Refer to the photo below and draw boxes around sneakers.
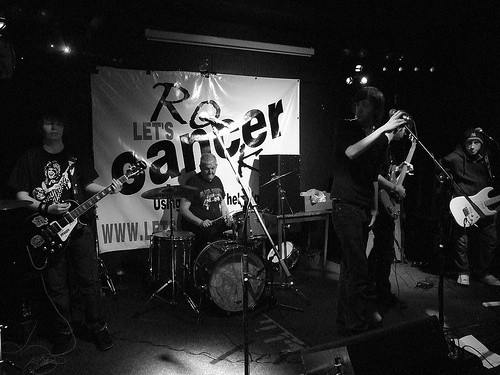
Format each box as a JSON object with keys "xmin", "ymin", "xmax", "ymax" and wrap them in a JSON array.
[
  {"xmin": 482, "ymin": 275, "xmax": 500, "ymax": 286},
  {"xmin": 457, "ymin": 274, "xmax": 469, "ymax": 285}
]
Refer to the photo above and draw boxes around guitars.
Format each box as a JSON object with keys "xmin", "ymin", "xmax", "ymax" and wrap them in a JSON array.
[
  {"xmin": 448, "ymin": 186, "xmax": 500, "ymax": 229},
  {"xmin": 22, "ymin": 160, "xmax": 147, "ymax": 259},
  {"xmin": 379, "ymin": 133, "xmax": 417, "ymax": 220}
]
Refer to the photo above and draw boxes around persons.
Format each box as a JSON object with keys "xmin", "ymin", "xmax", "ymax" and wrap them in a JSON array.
[
  {"xmin": 330, "ymin": 87, "xmax": 407, "ymax": 336},
  {"xmin": 435, "ymin": 130, "xmax": 500, "ymax": 286},
  {"xmin": 13, "ymin": 109, "xmax": 121, "ymax": 354},
  {"xmin": 368, "ymin": 115, "xmax": 417, "ymax": 310},
  {"xmin": 179, "ymin": 153, "xmax": 236, "ymax": 246}
]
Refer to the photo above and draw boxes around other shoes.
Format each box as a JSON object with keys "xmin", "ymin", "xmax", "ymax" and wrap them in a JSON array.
[
  {"xmin": 91, "ymin": 322, "xmax": 114, "ymax": 351},
  {"xmin": 50, "ymin": 331, "xmax": 79, "ymax": 356},
  {"xmin": 378, "ymin": 293, "xmax": 408, "ymax": 310}
]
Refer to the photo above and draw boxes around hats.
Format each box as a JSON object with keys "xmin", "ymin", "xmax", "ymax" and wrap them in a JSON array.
[{"xmin": 463, "ymin": 130, "xmax": 484, "ymax": 143}]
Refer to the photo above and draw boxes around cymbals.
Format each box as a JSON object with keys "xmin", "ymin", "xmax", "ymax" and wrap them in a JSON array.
[
  {"xmin": 260, "ymin": 170, "xmax": 295, "ymax": 189},
  {"xmin": 141, "ymin": 184, "xmax": 199, "ymax": 199},
  {"xmin": 1, "ymin": 198, "xmax": 33, "ymax": 210}
]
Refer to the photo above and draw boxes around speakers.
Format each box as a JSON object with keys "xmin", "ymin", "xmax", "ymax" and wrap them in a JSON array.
[
  {"xmin": 259, "ymin": 154, "xmax": 305, "ymax": 215},
  {"xmin": 301, "ymin": 314, "xmax": 459, "ymax": 375},
  {"xmin": 0, "ymin": 198, "xmax": 34, "ymax": 325}
]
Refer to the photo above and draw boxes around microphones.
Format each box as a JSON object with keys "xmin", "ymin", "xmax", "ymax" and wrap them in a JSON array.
[
  {"xmin": 475, "ymin": 128, "xmax": 494, "ymax": 141},
  {"xmin": 199, "ymin": 112, "xmax": 229, "ymax": 129},
  {"xmin": 389, "ymin": 109, "xmax": 411, "ymax": 120}
]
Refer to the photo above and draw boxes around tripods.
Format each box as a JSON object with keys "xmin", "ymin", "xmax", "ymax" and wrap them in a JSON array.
[
  {"xmin": 265, "ymin": 179, "xmax": 313, "ymax": 307},
  {"xmin": 132, "ymin": 197, "xmax": 204, "ymax": 326}
]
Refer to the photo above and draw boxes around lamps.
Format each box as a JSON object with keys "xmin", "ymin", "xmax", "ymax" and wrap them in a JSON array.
[{"xmin": 343, "ymin": 56, "xmax": 435, "ymax": 86}]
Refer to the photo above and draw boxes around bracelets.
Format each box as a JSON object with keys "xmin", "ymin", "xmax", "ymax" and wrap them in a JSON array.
[
  {"xmin": 44, "ymin": 203, "xmax": 51, "ymax": 211},
  {"xmin": 38, "ymin": 202, "xmax": 46, "ymax": 210}
]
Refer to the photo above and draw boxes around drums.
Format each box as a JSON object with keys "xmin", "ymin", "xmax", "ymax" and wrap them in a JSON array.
[
  {"xmin": 152, "ymin": 231, "xmax": 195, "ymax": 272},
  {"xmin": 267, "ymin": 240, "xmax": 299, "ymax": 273},
  {"xmin": 193, "ymin": 239, "xmax": 267, "ymax": 312},
  {"xmin": 233, "ymin": 209, "xmax": 268, "ymax": 241}
]
